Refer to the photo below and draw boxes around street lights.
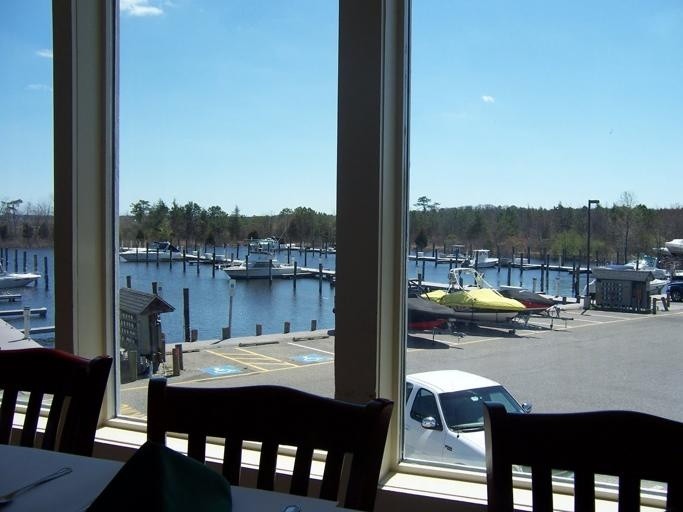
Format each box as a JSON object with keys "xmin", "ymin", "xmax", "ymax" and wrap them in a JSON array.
[{"xmin": 586, "ymin": 200, "xmax": 599, "ymax": 296}]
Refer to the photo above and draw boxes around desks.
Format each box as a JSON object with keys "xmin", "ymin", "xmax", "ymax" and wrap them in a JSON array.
[{"xmin": 2, "ymin": 445, "xmax": 353, "ymax": 511}]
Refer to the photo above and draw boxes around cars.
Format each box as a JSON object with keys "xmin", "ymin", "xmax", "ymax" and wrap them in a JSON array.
[{"xmin": 404, "ymin": 370, "xmax": 533, "ymax": 466}]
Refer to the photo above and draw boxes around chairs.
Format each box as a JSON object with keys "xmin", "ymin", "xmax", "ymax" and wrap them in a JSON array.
[
  {"xmin": 146, "ymin": 374, "xmax": 395, "ymax": 511},
  {"xmin": 0, "ymin": 346, "xmax": 115, "ymax": 457},
  {"xmin": 483, "ymin": 400, "xmax": 682, "ymax": 511}
]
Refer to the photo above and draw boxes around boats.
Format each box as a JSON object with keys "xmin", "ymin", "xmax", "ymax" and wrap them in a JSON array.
[
  {"xmin": 407, "ymin": 268, "xmax": 556, "ymax": 331},
  {"xmin": 0, "ymin": 274, "xmax": 42, "ymax": 290},
  {"xmin": 223, "ymin": 262, "xmax": 302, "ymax": 279},
  {"xmin": 469, "ymin": 249, "xmax": 499, "ymax": 268},
  {"xmin": 119, "ymin": 250, "xmax": 184, "ymax": 263}
]
